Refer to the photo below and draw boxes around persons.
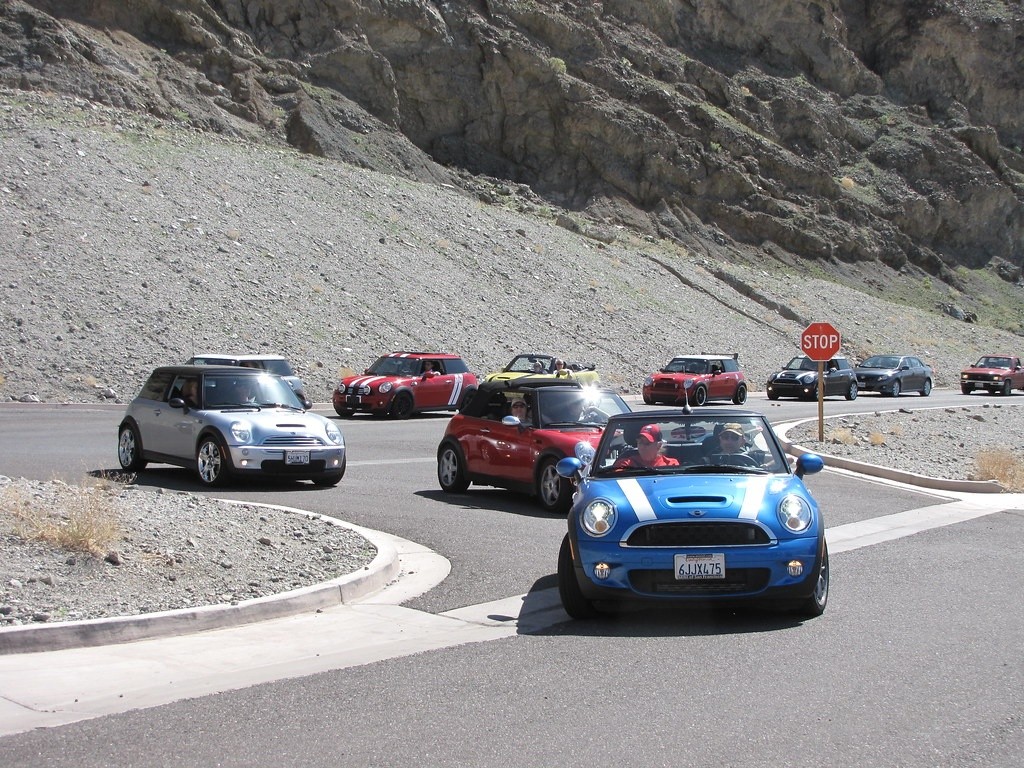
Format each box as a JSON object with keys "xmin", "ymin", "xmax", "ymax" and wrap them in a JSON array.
[
  {"xmin": 613, "ymin": 425, "xmax": 680, "ymax": 469},
  {"xmin": 232, "ymin": 384, "xmax": 257, "ymax": 402},
  {"xmin": 533, "ymin": 362, "xmax": 547, "ymax": 374},
  {"xmin": 700, "ymin": 423, "xmax": 750, "ymax": 466},
  {"xmin": 564, "ymin": 397, "xmax": 585, "ymax": 425},
  {"xmin": 419, "ymin": 361, "xmax": 442, "ymax": 376},
  {"xmin": 553, "ymin": 359, "xmax": 565, "ymax": 375},
  {"xmin": 511, "ymin": 397, "xmax": 528, "ymax": 423},
  {"xmin": 184, "ymin": 381, "xmax": 201, "ymax": 409}
]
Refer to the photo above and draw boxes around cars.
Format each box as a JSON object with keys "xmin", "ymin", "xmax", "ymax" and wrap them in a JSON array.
[
  {"xmin": 118, "ymin": 365, "xmax": 346, "ymax": 487},
  {"xmin": 436, "ymin": 378, "xmax": 634, "ymax": 517},
  {"xmin": 853, "ymin": 355, "xmax": 936, "ymax": 397},
  {"xmin": 333, "ymin": 352, "xmax": 477, "ymax": 418},
  {"xmin": 643, "ymin": 352, "xmax": 746, "ymax": 404},
  {"xmin": 557, "ymin": 392, "xmax": 829, "ymax": 616},
  {"xmin": 960, "ymin": 355, "xmax": 1024, "ymax": 397},
  {"xmin": 766, "ymin": 357, "xmax": 858, "ymax": 401},
  {"xmin": 185, "ymin": 354, "xmax": 305, "ymax": 410}
]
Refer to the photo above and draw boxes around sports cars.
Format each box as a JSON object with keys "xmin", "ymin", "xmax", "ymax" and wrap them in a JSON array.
[{"xmin": 482, "ymin": 354, "xmax": 601, "ymax": 388}]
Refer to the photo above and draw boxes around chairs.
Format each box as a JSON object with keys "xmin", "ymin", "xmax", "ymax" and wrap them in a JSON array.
[
  {"xmin": 701, "ymin": 424, "xmax": 722, "ymax": 456},
  {"xmin": 484, "ymin": 393, "xmax": 508, "ymax": 421},
  {"xmin": 711, "ymin": 365, "xmax": 718, "ymax": 373},
  {"xmin": 432, "ymin": 362, "xmax": 444, "ymax": 376},
  {"xmin": 409, "ymin": 362, "xmax": 418, "ymax": 372},
  {"xmin": 690, "ymin": 364, "xmax": 697, "ymax": 372},
  {"xmin": 618, "ymin": 424, "xmax": 640, "ymax": 458}
]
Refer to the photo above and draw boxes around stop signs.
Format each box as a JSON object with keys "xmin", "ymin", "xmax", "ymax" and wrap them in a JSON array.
[{"xmin": 799, "ymin": 322, "xmax": 840, "ymax": 363}]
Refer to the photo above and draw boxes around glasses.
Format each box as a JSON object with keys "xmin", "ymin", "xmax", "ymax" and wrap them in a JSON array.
[
  {"xmin": 534, "ymin": 365, "xmax": 540, "ymax": 368},
  {"xmin": 719, "ymin": 432, "xmax": 741, "ymax": 440},
  {"xmin": 512, "ymin": 404, "xmax": 524, "ymax": 409},
  {"xmin": 557, "ymin": 363, "xmax": 564, "ymax": 366},
  {"xmin": 424, "ymin": 363, "xmax": 431, "ymax": 366},
  {"xmin": 636, "ymin": 438, "xmax": 661, "ymax": 446}
]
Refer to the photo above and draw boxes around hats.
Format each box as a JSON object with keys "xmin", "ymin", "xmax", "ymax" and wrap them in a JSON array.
[
  {"xmin": 638, "ymin": 424, "xmax": 662, "ymax": 443},
  {"xmin": 718, "ymin": 423, "xmax": 745, "ymax": 439},
  {"xmin": 510, "ymin": 397, "xmax": 527, "ymax": 407}
]
